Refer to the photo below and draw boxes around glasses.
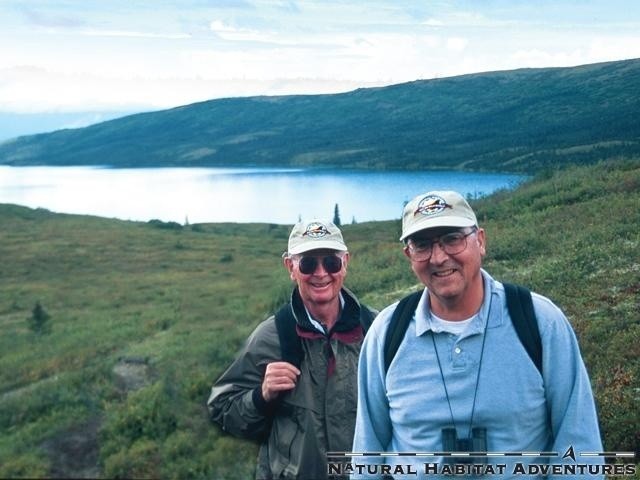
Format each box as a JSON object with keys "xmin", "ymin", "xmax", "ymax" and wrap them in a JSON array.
[
  {"xmin": 406, "ymin": 229, "xmax": 476, "ymax": 261},
  {"xmin": 291, "ymin": 256, "xmax": 344, "ymax": 274}
]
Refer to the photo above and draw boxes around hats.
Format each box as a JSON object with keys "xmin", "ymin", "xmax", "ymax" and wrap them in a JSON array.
[
  {"xmin": 398, "ymin": 190, "xmax": 478, "ymax": 244},
  {"xmin": 287, "ymin": 216, "xmax": 348, "ymax": 256}
]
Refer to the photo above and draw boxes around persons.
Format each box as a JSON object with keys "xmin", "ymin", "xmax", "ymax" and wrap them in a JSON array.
[
  {"xmin": 206, "ymin": 216, "xmax": 381, "ymax": 480},
  {"xmin": 351, "ymin": 192, "xmax": 605, "ymax": 480}
]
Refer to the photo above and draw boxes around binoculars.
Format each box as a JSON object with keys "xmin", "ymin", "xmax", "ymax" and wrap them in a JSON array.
[{"xmin": 442, "ymin": 427, "xmax": 488, "ymax": 476}]
